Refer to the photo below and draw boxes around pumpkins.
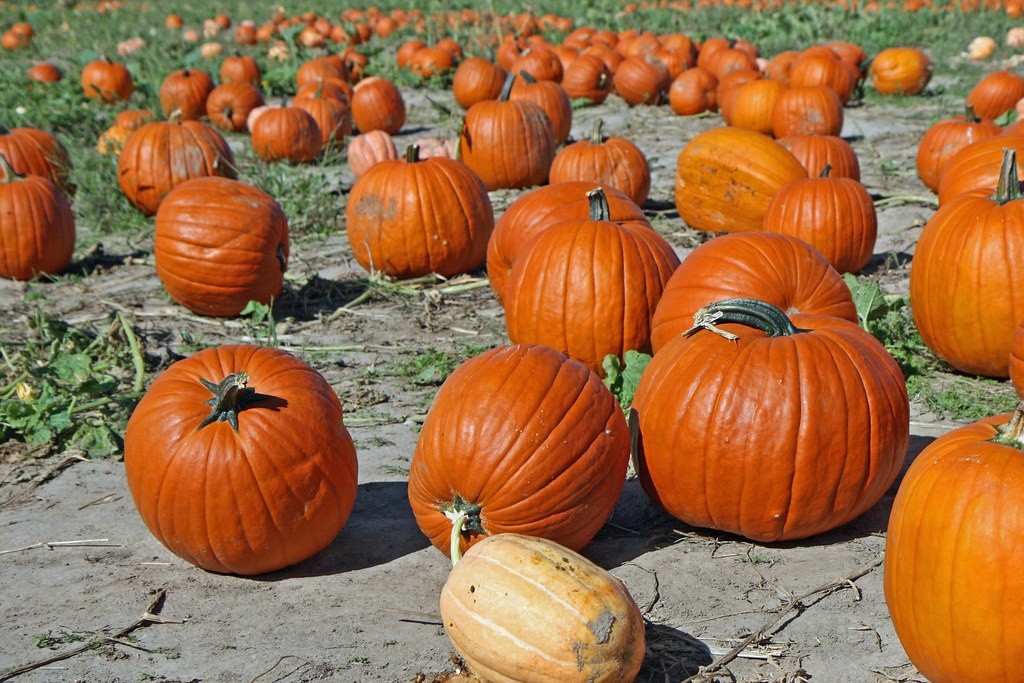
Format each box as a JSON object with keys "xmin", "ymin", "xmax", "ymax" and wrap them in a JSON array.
[{"xmin": 0, "ymin": 0, "xmax": 1024, "ymax": 683}]
